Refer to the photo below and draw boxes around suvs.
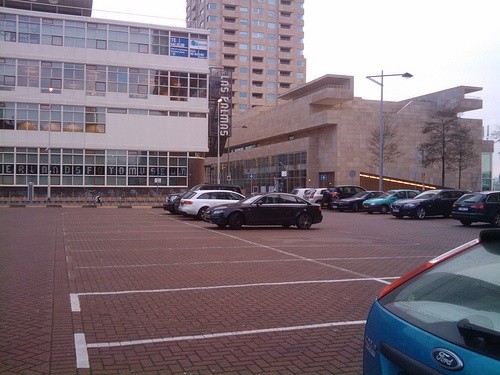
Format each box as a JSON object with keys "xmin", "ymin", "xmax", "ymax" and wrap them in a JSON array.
[{"xmin": 162, "ymin": 184, "xmax": 241, "ymax": 214}]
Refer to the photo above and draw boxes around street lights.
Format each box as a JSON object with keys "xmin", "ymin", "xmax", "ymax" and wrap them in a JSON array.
[
  {"xmin": 216, "ymin": 97, "xmax": 225, "ymax": 182},
  {"xmin": 226, "ymin": 125, "xmax": 248, "ymax": 184},
  {"xmin": 366, "ymin": 71, "xmax": 414, "ymax": 190}
]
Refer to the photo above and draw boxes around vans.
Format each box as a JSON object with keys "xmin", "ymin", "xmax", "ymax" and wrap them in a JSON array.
[{"xmin": 323, "ymin": 185, "xmax": 367, "ymax": 210}]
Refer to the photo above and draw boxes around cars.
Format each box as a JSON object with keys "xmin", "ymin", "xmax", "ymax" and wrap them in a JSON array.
[
  {"xmin": 205, "ymin": 193, "xmax": 323, "ymax": 230},
  {"xmin": 389, "ymin": 189, "xmax": 473, "ymax": 220},
  {"xmin": 291, "ymin": 188, "xmax": 311, "ymax": 200},
  {"xmin": 361, "ymin": 189, "xmax": 421, "ymax": 214},
  {"xmin": 331, "ymin": 191, "xmax": 390, "ymax": 213},
  {"xmin": 303, "ymin": 187, "xmax": 328, "ymax": 209},
  {"xmin": 178, "ymin": 189, "xmax": 246, "ymax": 222},
  {"xmin": 362, "ymin": 215, "xmax": 500, "ymax": 375},
  {"xmin": 451, "ymin": 190, "xmax": 500, "ymax": 228}
]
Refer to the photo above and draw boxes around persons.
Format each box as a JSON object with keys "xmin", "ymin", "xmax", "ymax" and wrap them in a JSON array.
[{"xmin": 322, "ymin": 182, "xmax": 340, "ymax": 209}]
